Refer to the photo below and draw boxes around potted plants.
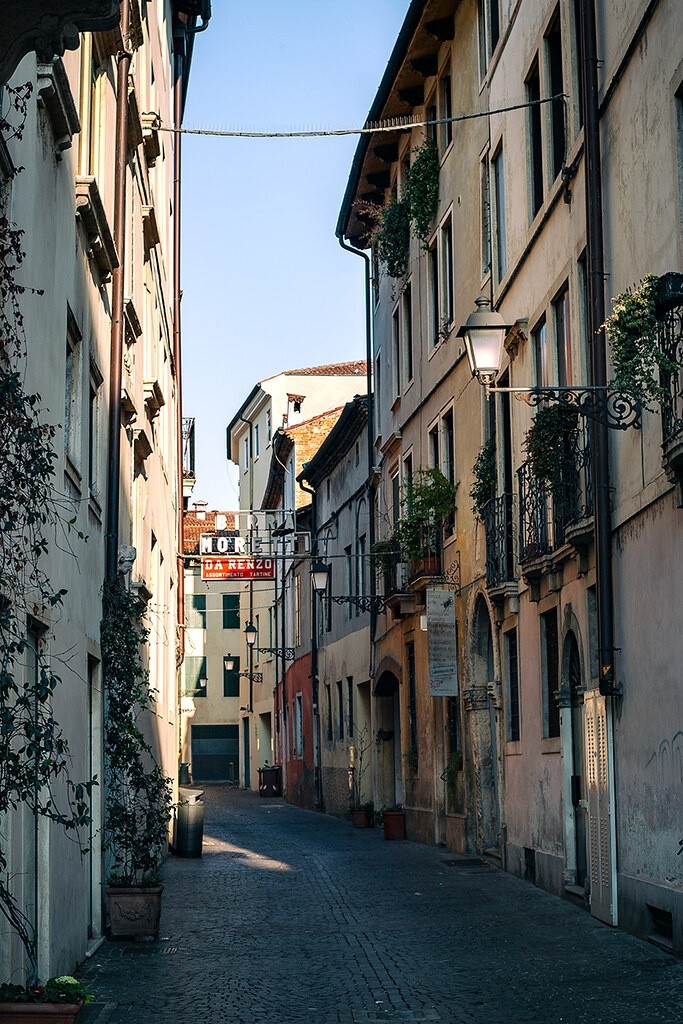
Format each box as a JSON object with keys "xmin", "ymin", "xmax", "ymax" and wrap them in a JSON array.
[
  {"xmin": 381, "ymin": 804, "xmax": 406, "ymax": 841},
  {"xmin": 100, "ymin": 777, "xmax": 177, "ymax": 939},
  {"xmin": 343, "ymin": 724, "xmax": 377, "ymax": 829}
]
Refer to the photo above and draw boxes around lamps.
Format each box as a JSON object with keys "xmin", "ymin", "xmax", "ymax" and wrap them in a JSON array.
[
  {"xmin": 180, "ymin": 674, "xmax": 208, "ymax": 696},
  {"xmin": 242, "ymin": 621, "xmax": 296, "ymax": 661},
  {"xmin": 455, "ymin": 295, "xmax": 644, "ymax": 433},
  {"xmin": 308, "ymin": 557, "xmax": 390, "ymax": 616},
  {"xmin": 224, "ymin": 653, "xmax": 263, "ymax": 683}
]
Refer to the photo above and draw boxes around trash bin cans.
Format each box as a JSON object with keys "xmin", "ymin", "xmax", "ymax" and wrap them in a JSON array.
[
  {"xmin": 179, "ymin": 762, "xmax": 191, "ymax": 785},
  {"xmin": 177, "ymin": 789, "xmax": 205, "ymax": 858},
  {"xmin": 256, "ymin": 764, "xmax": 282, "ymax": 798}
]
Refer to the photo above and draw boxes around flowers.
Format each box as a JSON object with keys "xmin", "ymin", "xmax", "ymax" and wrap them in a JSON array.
[{"xmin": 1, "ymin": 967, "xmax": 103, "ymax": 1007}]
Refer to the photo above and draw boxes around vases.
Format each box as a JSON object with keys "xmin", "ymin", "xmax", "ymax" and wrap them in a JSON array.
[{"xmin": 0, "ymin": 1000, "xmax": 82, "ymax": 1024}]
